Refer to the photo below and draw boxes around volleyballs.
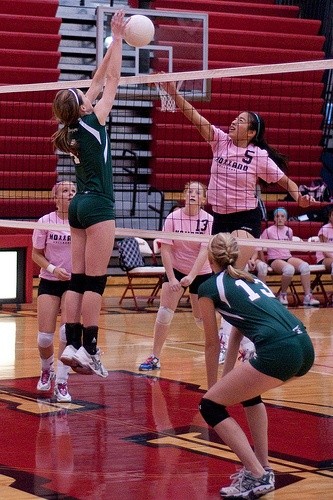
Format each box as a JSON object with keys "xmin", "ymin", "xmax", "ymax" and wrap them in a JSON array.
[{"xmin": 123, "ymin": 15, "xmax": 155, "ymax": 47}]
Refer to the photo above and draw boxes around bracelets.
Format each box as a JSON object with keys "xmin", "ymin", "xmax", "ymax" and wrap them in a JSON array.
[{"xmin": 46, "ymin": 264, "xmax": 57, "ymax": 275}]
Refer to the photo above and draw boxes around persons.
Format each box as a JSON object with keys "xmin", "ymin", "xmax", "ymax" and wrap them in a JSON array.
[
  {"xmin": 31, "ymin": 181, "xmax": 76, "ymax": 401},
  {"xmin": 316, "ymin": 208, "xmax": 333, "ymax": 279},
  {"xmin": 198, "ymin": 232, "xmax": 315, "ymax": 499},
  {"xmin": 244, "ymin": 248, "xmax": 267, "ymax": 284},
  {"xmin": 156, "ymin": 71, "xmax": 320, "ymax": 365},
  {"xmin": 260, "ymin": 208, "xmax": 320, "ymax": 306},
  {"xmin": 138, "ymin": 182, "xmax": 214, "ymax": 370},
  {"xmin": 52, "ymin": 10, "xmax": 131, "ymax": 378}
]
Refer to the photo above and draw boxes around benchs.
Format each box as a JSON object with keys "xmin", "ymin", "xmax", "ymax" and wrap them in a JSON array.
[
  {"xmin": 0, "ymin": 0, "xmax": 59, "ymax": 219},
  {"xmin": 128, "ymin": 0, "xmax": 333, "ymax": 267}
]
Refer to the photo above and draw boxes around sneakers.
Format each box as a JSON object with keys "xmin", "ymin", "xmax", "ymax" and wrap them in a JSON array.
[
  {"xmin": 279, "ymin": 291, "xmax": 289, "ymax": 305},
  {"xmin": 60, "ymin": 345, "xmax": 95, "ymax": 375},
  {"xmin": 73, "ymin": 346, "xmax": 108, "ymax": 378},
  {"xmin": 239, "ymin": 351, "xmax": 257, "ymax": 363},
  {"xmin": 219, "ymin": 334, "xmax": 229, "ymax": 364},
  {"xmin": 139, "ymin": 356, "xmax": 160, "ymax": 370},
  {"xmin": 219, "ymin": 468, "xmax": 275, "ymax": 500},
  {"xmin": 303, "ymin": 295, "xmax": 320, "ymax": 305},
  {"xmin": 36, "ymin": 366, "xmax": 56, "ymax": 391},
  {"xmin": 53, "ymin": 379, "xmax": 71, "ymax": 402}
]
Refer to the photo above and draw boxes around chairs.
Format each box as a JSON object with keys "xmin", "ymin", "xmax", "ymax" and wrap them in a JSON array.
[{"xmin": 117, "ymin": 233, "xmax": 333, "ymax": 308}]
